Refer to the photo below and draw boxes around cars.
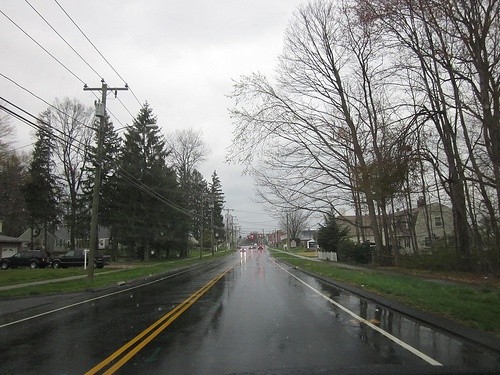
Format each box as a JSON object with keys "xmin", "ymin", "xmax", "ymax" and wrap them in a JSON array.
[
  {"xmin": 258, "ymin": 245, "xmax": 263, "ymax": 250},
  {"xmin": 240, "ymin": 247, "xmax": 247, "ymax": 252}
]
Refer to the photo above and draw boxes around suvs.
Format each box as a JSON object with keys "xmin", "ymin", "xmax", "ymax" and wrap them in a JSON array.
[
  {"xmin": 0, "ymin": 250, "xmax": 49, "ymax": 269},
  {"xmin": 52, "ymin": 248, "xmax": 104, "ymax": 269}
]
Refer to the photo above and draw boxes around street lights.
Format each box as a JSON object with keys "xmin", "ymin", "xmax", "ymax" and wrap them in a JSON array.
[{"xmin": 80, "ymin": 151, "xmax": 103, "ymax": 281}]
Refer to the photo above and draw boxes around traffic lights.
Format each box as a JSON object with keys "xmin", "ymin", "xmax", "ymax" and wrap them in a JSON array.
[{"xmin": 252, "ymin": 235, "xmax": 254, "ymax": 239}]
